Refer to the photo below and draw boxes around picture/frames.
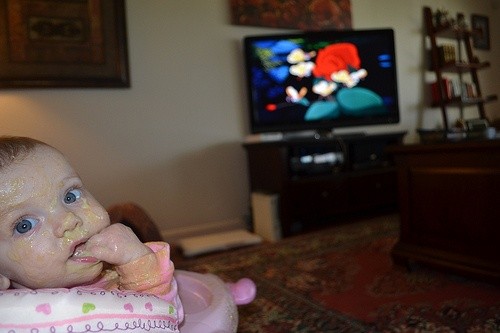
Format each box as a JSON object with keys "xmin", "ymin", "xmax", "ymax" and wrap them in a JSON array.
[
  {"xmin": 470, "ymin": 13, "xmax": 490, "ymax": 50},
  {"xmin": 0, "ymin": 0, "xmax": 130, "ymax": 89}
]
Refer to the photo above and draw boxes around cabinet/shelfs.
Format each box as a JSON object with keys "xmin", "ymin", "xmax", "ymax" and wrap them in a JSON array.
[
  {"xmin": 424, "ymin": 6, "xmax": 500, "ymax": 142},
  {"xmin": 243, "ymin": 131, "xmax": 410, "ymax": 238}
]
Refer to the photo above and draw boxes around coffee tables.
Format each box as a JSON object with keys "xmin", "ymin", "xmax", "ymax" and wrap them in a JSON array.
[{"xmin": 387, "ymin": 139, "xmax": 500, "ymax": 283}]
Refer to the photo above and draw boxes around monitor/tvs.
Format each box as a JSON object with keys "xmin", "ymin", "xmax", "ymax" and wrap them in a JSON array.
[{"xmin": 244, "ymin": 27, "xmax": 400, "ymax": 141}]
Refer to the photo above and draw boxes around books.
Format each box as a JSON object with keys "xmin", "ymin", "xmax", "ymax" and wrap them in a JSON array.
[
  {"xmin": 437, "ymin": 45, "xmax": 456, "ymax": 62},
  {"xmin": 432, "ymin": 80, "xmax": 474, "ymax": 104}
]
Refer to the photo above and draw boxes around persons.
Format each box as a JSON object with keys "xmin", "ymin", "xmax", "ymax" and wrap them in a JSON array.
[{"xmin": 0, "ymin": 135, "xmax": 185, "ymax": 333}]
